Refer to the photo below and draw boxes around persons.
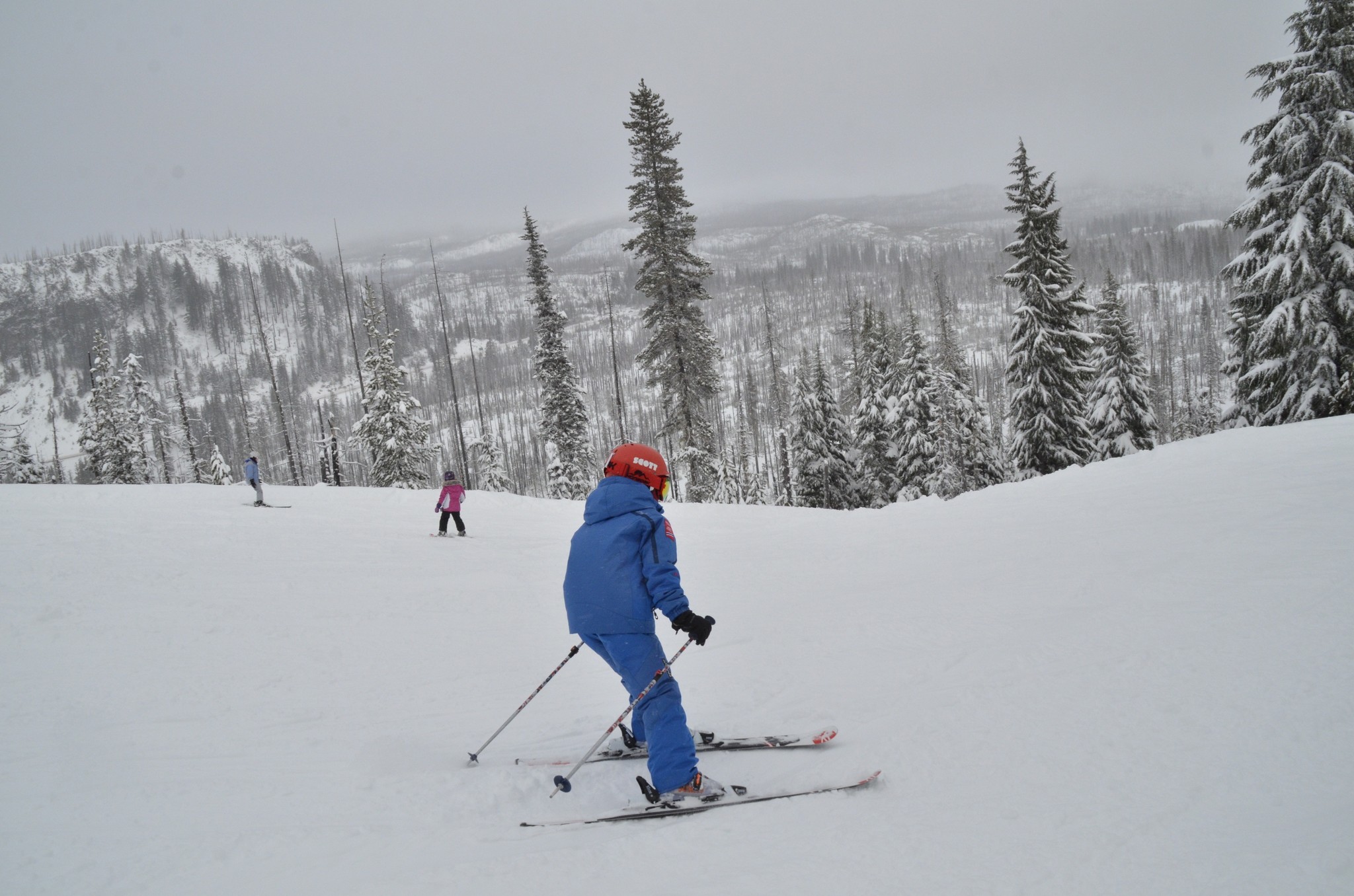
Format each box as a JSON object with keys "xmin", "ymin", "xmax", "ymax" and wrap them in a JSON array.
[
  {"xmin": 435, "ymin": 471, "xmax": 466, "ymax": 536},
  {"xmin": 563, "ymin": 443, "xmax": 723, "ymax": 797},
  {"xmin": 244, "ymin": 451, "xmax": 267, "ymax": 507}
]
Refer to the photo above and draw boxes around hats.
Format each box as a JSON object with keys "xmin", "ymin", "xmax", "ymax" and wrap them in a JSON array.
[{"xmin": 250, "ymin": 452, "xmax": 260, "ymax": 458}]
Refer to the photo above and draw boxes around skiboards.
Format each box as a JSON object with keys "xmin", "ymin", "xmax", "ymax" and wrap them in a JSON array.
[
  {"xmin": 429, "ymin": 531, "xmax": 476, "ymax": 538},
  {"xmin": 517, "ymin": 725, "xmax": 883, "ymax": 829},
  {"xmin": 241, "ymin": 502, "xmax": 292, "ymax": 508}
]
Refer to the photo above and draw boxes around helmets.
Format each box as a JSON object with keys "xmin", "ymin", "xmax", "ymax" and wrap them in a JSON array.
[
  {"xmin": 604, "ymin": 444, "xmax": 669, "ymax": 502},
  {"xmin": 444, "ymin": 471, "xmax": 455, "ymax": 480}
]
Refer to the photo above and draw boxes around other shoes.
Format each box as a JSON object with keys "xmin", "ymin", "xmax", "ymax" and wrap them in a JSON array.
[
  {"xmin": 436, "ymin": 531, "xmax": 447, "ymax": 537},
  {"xmin": 456, "ymin": 531, "xmax": 465, "ymax": 536}
]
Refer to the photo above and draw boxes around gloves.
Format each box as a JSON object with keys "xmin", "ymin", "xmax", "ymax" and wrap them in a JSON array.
[
  {"xmin": 250, "ymin": 479, "xmax": 257, "ymax": 488},
  {"xmin": 435, "ymin": 507, "xmax": 439, "ymax": 513},
  {"xmin": 672, "ymin": 611, "xmax": 715, "ymax": 648},
  {"xmin": 259, "ymin": 479, "xmax": 262, "ymax": 483}
]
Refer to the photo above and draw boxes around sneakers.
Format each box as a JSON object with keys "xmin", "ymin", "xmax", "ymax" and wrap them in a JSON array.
[
  {"xmin": 658, "ymin": 770, "xmax": 723, "ymax": 796},
  {"xmin": 255, "ymin": 502, "xmax": 269, "ymax": 507}
]
